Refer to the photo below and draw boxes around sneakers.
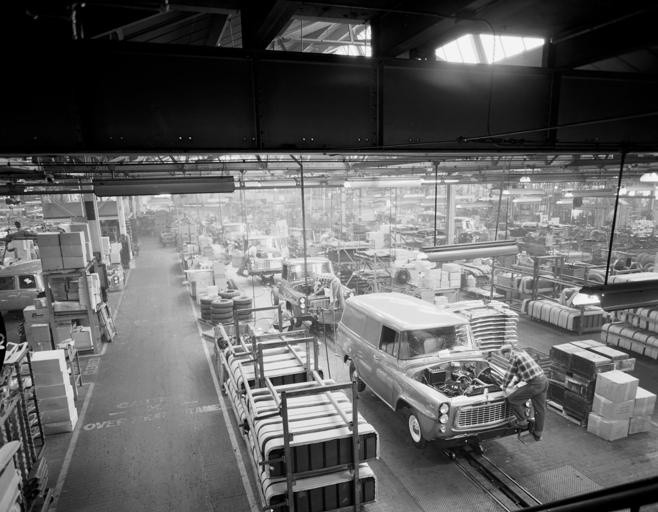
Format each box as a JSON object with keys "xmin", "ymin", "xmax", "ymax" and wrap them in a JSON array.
[
  {"xmin": 529, "ymin": 423, "xmax": 540, "ymax": 441},
  {"xmin": 511, "ymin": 420, "xmax": 529, "ymax": 430}
]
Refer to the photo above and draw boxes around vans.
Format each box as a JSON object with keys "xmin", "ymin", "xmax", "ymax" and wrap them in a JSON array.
[{"xmin": 0, "ymin": 260, "xmax": 46, "ymax": 316}]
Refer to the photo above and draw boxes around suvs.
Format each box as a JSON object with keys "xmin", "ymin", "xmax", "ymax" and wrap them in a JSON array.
[
  {"xmin": 202, "ymin": 211, "xmax": 353, "ymax": 333},
  {"xmin": 334, "ymin": 289, "xmax": 536, "ymax": 453}
]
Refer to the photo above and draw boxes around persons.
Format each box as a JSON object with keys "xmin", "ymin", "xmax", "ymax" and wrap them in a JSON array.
[
  {"xmin": 310, "ymin": 272, "xmax": 345, "ymax": 313},
  {"xmin": 237, "ymin": 240, "xmax": 260, "ymax": 276},
  {"xmin": 498, "ymin": 343, "xmax": 550, "ymax": 441},
  {"xmin": 517, "ymin": 249, "xmax": 534, "ymax": 267}
]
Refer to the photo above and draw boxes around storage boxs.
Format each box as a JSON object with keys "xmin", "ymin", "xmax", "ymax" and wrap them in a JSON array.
[
  {"xmin": 182, "ymin": 261, "xmax": 231, "ymax": 305},
  {"xmin": 427, "ymin": 262, "xmax": 463, "ymax": 293},
  {"xmin": 17, "ymin": 221, "xmax": 123, "ymax": 435},
  {"xmin": 539, "ymin": 335, "xmax": 657, "ymax": 445}
]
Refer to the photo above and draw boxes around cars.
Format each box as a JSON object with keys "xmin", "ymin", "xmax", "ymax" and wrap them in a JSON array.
[{"xmin": 455, "ymin": 218, "xmax": 489, "ymax": 240}]
